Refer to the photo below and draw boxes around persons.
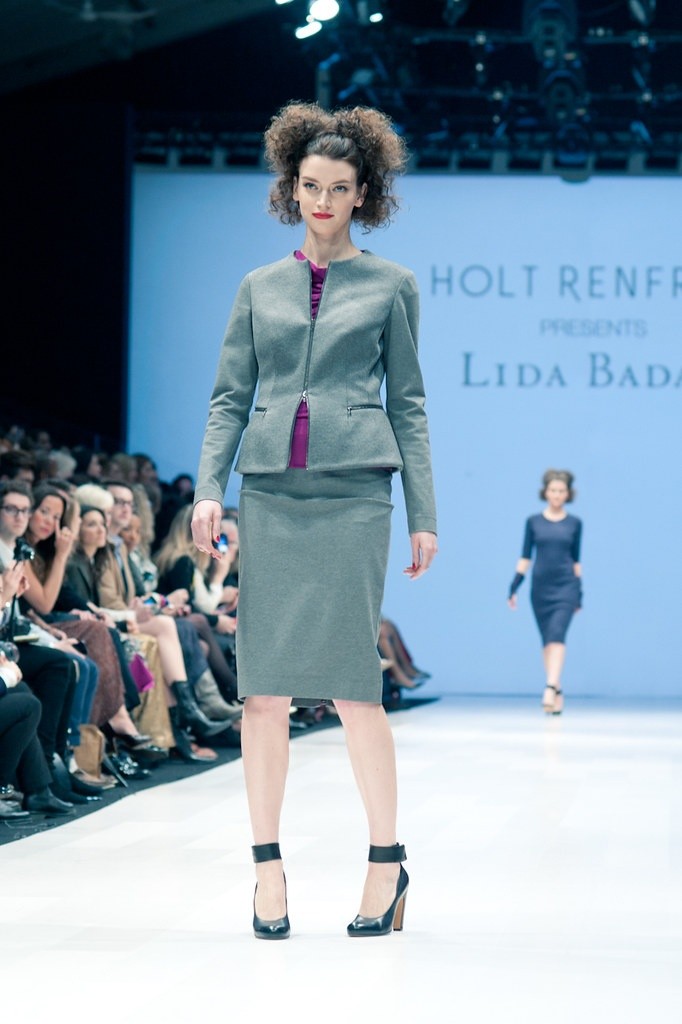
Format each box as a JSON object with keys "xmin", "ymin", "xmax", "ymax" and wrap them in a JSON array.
[
  {"xmin": 191, "ymin": 105, "xmax": 440, "ymax": 938},
  {"xmin": 508, "ymin": 470, "xmax": 582, "ymax": 717},
  {"xmin": 0, "ymin": 427, "xmax": 430, "ymax": 817}
]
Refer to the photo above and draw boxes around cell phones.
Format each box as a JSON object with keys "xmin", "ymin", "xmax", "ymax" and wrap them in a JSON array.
[
  {"xmin": 142, "ymin": 596, "xmax": 157, "ymax": 605},
  {"xmin": 73, "ymin": 640, "xmax": 89, "ymax": 655},
  {"xmin": 213, "ymin": 532, "xmax": 229, "ymax": 554}
]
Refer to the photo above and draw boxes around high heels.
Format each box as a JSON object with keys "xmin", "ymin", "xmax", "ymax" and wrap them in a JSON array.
[
  {"xmin": 347, "ymin": 842, "xmax": 409, "ymax": 936},
  {"xmin": 251, "ymin": 843, "xmax": 290, "ymax": 938}
]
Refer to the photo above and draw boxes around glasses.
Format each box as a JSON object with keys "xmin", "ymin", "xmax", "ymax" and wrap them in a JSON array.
[
  {"xmin": 0, "ymin": 504, "xmax": 33, "ymax": 517},
  {"xmin": 112, "ymin": 498, "xmax": 134, "ymax": 508}
]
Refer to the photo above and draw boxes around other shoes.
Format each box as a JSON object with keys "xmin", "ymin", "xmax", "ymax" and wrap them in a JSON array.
[
  {"xmin": 540, "ymin": 684, "xmax": 564, "ymax": 715},
  {"xmin": 0, "ymin": 722, "xmax": 168, "ymax": 822}
]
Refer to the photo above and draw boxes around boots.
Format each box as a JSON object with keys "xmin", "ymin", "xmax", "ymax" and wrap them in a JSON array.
[{"xmin": 166, "ymin": 671, "xmax": 245, "ymax": 763}]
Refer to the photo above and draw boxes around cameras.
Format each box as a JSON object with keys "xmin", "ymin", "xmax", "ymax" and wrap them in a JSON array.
[
  {"xmin": 0, "ymin": 641, "xmax": 19, "ymax": 664},
  {"xmin": 13, "ymin": 539, "xmax": 34, "ymax": 561}
]
